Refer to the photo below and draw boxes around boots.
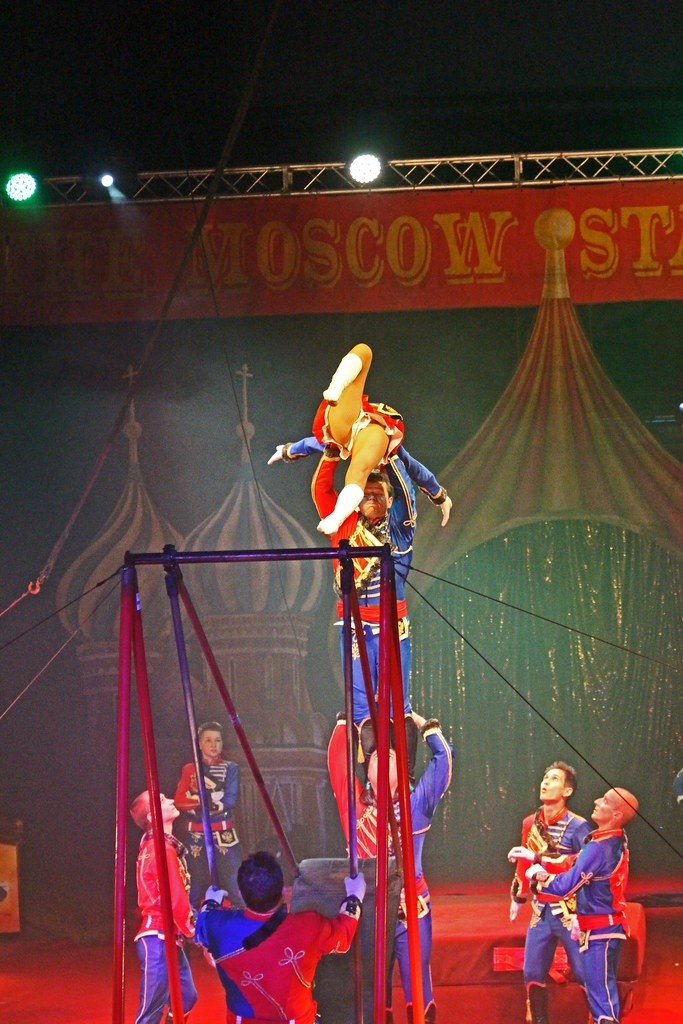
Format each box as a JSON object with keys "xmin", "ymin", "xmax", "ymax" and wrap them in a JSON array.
[
  {"xmin": 406, "ymin": 1000, "xmax": 437, "ymax": 1024},
  {"xmin": 593, "ymin": 1015, "xmax": 619, "ymax": 1024},
  {"xmin": 352, "ymin": 714, "xmax": 418, "ymax": 805},
  {"xmin": 384, "ymin": 1007, "xmax": 394, "ymax": 1024},
  {"xmin": 166, "ymin": 1012, "xmax": 189, "ymax": 1024},
  {"xmin": 526, "ymin": 981, "xmax": 546, "ymax": 1024}
]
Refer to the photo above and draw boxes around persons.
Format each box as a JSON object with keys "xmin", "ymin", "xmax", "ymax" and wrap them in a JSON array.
[
  {"xmin": 128, "ymin": 790, "xmax": 198, "ymax": 1024},
  {"xmin": 310, "ymin": 443, "xmax": 417, "ymax": 804},
  {"xmin": 267, "ymin": 343, "xmax": 453, "ymax": 535},
  {"xmin": 510, "ymin": 761, "xmax": 595, "ymax": 1024},
  {"xmin": 507, "ymin": 787, "xmax": 639, "ymax": 1024},
  {"xmin": 194, "ymin": 851, "xmax": 367, "ymax": 1024},
  {"xmin": 174, "ymin": 721, "xmax": 247, "ymax": 913},
  {"xmin": 675, "ymin": 767, "xmax": 683, "ymax": 805},
  {"xmin": 327, "ymin": 709, "xmax": 456, "ymax": 1024}
]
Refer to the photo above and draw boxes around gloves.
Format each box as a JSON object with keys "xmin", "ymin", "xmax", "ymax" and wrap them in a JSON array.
[
  {"xmin": 525, "ymin": 864, "xmax": 546, "ymax": 879},
  {"xmin": 211, "ymin": 790, "xmax": 224, "ymax": 801},
  {"xmin": 510, "ymin": 901, "xmax": 521, "ymax": 923},
  {"xmin": 404, "ymin": 710, "xmax": 427, "ymax": 732},
  {"xmin": 344, "ymin": 872, "xmax": 367, "ymax": 904},
  {"xmin": 568, "ymin": 915, "xmax": 581, "ymax": 942},
  {"xmin": 204, "ymin": 885, "xmax": 229, "ymax": 905},
  {"xmin": 440, "ymin": 496, "xmax": 453, "ymax": 528},
  {"xmin": 507, "ymin": 846, "xmax": 534, "ymax": 864},
  {"xmin": 267, "ymin": 445, "xmax": 284, "ymax": 465}
]
